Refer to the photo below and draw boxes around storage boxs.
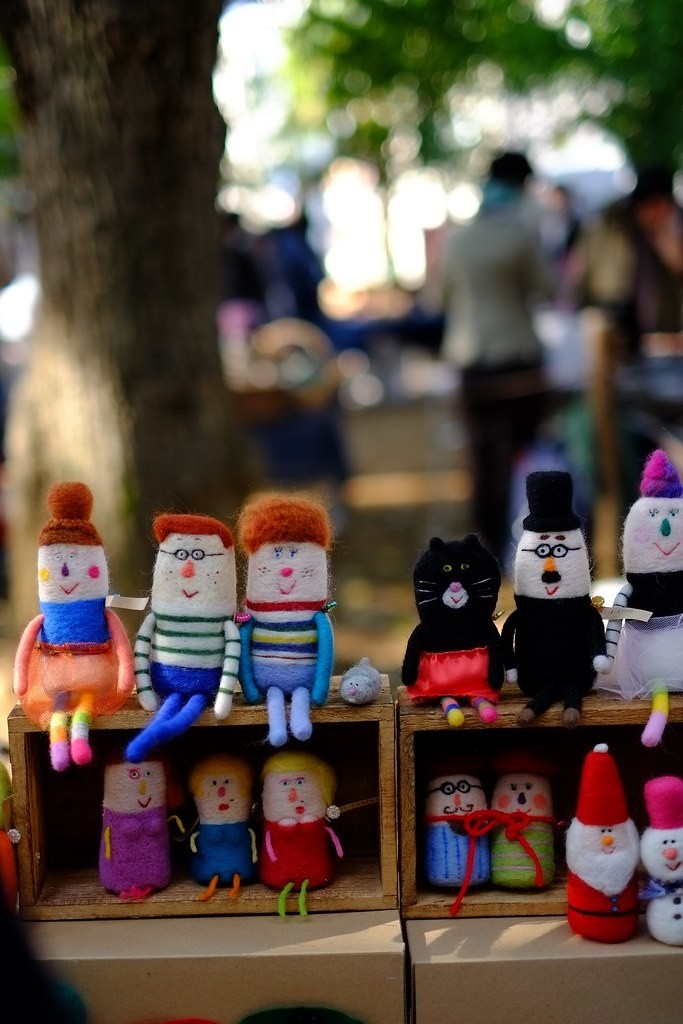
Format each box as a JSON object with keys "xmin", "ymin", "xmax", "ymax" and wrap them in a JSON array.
[{"xmin": 4, "ymin": 675, "xmax": 683, "ymax": 1024}]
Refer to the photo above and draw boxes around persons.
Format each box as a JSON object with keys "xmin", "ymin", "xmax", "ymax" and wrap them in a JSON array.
[
  {"xmin": 206, "ymin": 213, "xmax": 447, "ymax": 501},
  {"xmin": 437, "ymin": 153, "xmax": 558, "ymax": 532},
  {"xmin": 544, "ymin": 176, "xmax": 587, "ymax": 261},
  {"xmin": 588, "ymin": 124, "xmax": 682, "ymax": 573}
]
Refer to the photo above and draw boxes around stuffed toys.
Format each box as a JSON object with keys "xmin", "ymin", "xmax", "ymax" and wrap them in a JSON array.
[
  {"xmin": 565, "ymin": 747, "xmax": 641, "ymax": 944},
  {"xmin": 338, "ymin": 657, "xmax": 381, "ymax": 705},
  {"xmin": 126, "ymin": 514, "xmax": 241, "ymax": 762},
  {"xmin": 236, "ymin": 490, "xmax": 335, "ymax": 744},
  {"xmin": 253, "ymin": 753, "xmax": 344, "ymax": 918},
  {"xmin": 191, "ymin": 752, "xmax": 257, "ymax": 899},
  {"xmin": 425, "ymin": 742, "xmax": 491, "ymax": 889},
  {"xmin": 485, "ymin": 747, "xmax": 555, "ymax": 887},
  {"xmin": 593, "ymin": 450, "xmax": 683, "ymax": 747},
  {"xmin": 401, "ymin": 534, "xmax": 502, "ymax": 725},
  {"xmin": 13, "ymin": 482, "xmax": 135, "ymax": 770},
  {"xmin": 639, "ymin": 777, "xmax": 683, "ymax": 948},
  {"xmin": 99, "ymin": 762, "xmax": 183, "ymax": 901},
  {"xmin": 502, "ymin": 472, "xmax": 606, "ymax": 726}
]
